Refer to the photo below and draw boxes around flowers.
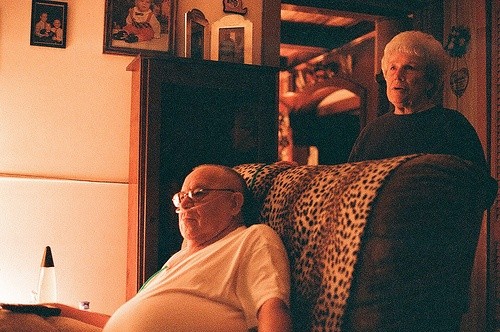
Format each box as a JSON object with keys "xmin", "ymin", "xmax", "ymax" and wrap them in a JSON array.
[{"xmin": 442, "ymin": 24, "xmax": 471, "ymax": 56}]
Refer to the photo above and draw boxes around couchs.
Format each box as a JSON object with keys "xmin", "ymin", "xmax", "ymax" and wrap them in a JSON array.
[{"xmin": 231, "ymin": 154, "xmax": 498, "ymax": 332}]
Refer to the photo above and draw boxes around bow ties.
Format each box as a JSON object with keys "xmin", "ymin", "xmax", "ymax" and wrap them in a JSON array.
[{"xmin": 135, "ymin": 11, "xmax": 144, "ymax": 16}]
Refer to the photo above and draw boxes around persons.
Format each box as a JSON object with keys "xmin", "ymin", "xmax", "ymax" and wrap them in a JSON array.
[
  {"xmin": 50, "ymin": 19, "xmax": 63, "ymax": 44},
  {"xmin": 113, "ymin": 0, "xmax": 161, "ymax": 43},
  {"xmin": 35, "ymin": 12, "xmax": 51, "ymax": 42},
  {"xmin": 33, "ymin": 163, "xmax": 292, "ymax": 332},
  {"xmin": 348, "ymin": 32, "xmax": 498, "ymax": 213}
]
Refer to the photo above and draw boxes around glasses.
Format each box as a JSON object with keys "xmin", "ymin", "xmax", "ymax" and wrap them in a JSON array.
[{"xmin": 171, "ymin": 187, "xmax": 236, "ymax": 207}]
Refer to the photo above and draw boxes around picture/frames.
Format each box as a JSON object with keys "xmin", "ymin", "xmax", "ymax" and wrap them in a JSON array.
[
  {"xmin": 29, "ymin": 0, "xmax": 67, "ymax": 48},
  {"xmin": 184, "ymin": 9, "xmax": 210, "ymax": 59},
  {"xmin": 103, "ymin": 0, "xmax": 179, "ymax": 58},
  {"xmin": 210, "ymin": 15, "xmax": 252, "ymax": 65}
]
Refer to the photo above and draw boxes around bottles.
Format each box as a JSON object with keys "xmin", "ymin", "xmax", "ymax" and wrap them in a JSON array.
[{"xmin": 36, "ymin": 245, "xmax": 59, "ymax": 305}]
